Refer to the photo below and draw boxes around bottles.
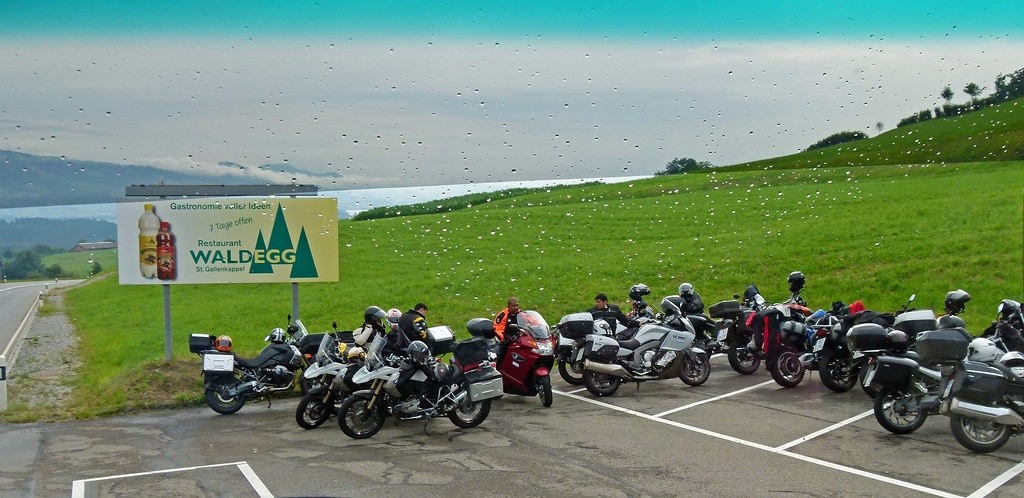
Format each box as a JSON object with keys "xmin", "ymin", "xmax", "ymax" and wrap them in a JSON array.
[
  {"xmin": 137, "ymin": 204, "xmax": 160, "ymax": 278},
  {"xmin": 156, "ymin": 222, "xmax": 176, "ymax": 279}
]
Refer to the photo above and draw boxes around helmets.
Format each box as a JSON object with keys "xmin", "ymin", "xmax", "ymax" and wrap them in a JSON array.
[
  {"xmin": 628, "ymin": 283, "xmax": 651, "ymax": 302},
  {"xmin": 943, "ymin": 289, "xmax": 971, "ymax": 316},
  {"xmin": 269, "ymin": 328, "xmax": 286, "ymax": 344},
  {"xmin": 886, "ymin": 330, "xmax": 909, "ymax": 352},
  {"xmin": 967, "ymin": 337, "xmax": 997, "ymax": 362},
  {"xmin": 998, "ymin": 350, "xmax": 1024, "ymax": 379},
  {"xmin": 678, "ymin": 283, "xmax": 695, "ymax": 297},
  {"xmin": 215, "ymin": 335, "xmax": 233, "ymax": 352},
  {"xmin": 1001, "ymin": 299, "xmax": 1021, "ymax": 319},
  {"xmin": 406, "ymin": 340, "xmax": 431, "ymax": 365},
  {"xmin": 364, "ymin": 305, "xmax": 385, "ymax": 332},
  {"xmin": 592, "ymin": 319, "xmax": 613, "ymax": 337},
  {"xmin": 384, "ymin": 307, "xmax": 403, "ymax": 329},
  {"xmin": 786, "ymin": 271, "xmax": 806, "ymax": 293}
]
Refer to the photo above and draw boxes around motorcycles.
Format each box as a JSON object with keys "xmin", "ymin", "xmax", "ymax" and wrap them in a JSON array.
[
  {"xmin": 467, "ymin": 310, "xmax": 563, "ymax": 408},
  {"xmin": 557, "ymin": 297, "xmax": 711, "ymax": 398},
  {"xmin": 337, "ymin": 316, "xmax": 505, "ymax": 440},
  {"xmin": 845, "ymin": 302, "xmax": 971, "ymax": 435},
  {"xmin": 189, "ymin": 314, "xmax": 319, "ymax": 415},
  {"xmin": 913, "ymin": 299, "xmax": 1024, "ymax": 454},
  {"xmin": 293, "ymin": 287, "xmax": 919, "ymax": 430}
]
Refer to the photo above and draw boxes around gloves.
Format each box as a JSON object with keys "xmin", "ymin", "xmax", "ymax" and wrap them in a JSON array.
[{"xmin": 365, "ymin": 323, "xmax": 373, "ymax": 332}]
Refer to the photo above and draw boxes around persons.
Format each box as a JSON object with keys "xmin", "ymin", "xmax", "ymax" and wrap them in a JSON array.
[
  {"xmin": 586, "ymin": 294, "xmax": 645, "ymax": 339},
  {"xmin": 494, "ymin": 297, "xmax": 520, "ymax": 369},
  {"xmin": 399, "ymin": 302, "xmax": 433, "ymax": 348},
  {"xmin": 353, "ymin": 306, "xmax": 401, "ymax": 368}
]
